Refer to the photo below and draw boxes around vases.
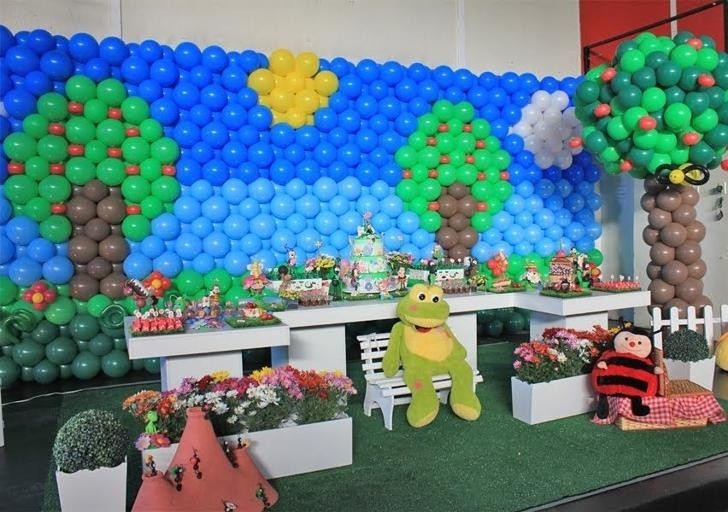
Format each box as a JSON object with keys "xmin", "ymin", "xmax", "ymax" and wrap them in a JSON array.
[
  {"xmin": 508, "ymin": 374, "xmax": 615, "ymax": 426},
  {"xmin": 136, "ymin": 418, "xmax": 358, "ymax": 479}
]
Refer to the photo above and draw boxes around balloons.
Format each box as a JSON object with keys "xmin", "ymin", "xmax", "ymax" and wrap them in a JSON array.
[{"xmin": 2, "ymin": 24, "xmax": 726, "ymax": 389}]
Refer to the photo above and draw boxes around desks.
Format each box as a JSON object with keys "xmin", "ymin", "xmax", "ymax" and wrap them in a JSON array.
[{"xmin": 123, "ymin": 275, "xmax": 653, "ymax": 393}]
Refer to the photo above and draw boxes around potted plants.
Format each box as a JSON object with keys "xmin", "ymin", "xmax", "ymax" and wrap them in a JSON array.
[
  {"xmin": 53, "ymin": 406, "xmax": 135, "ymax": 512},
  {"xmin": 665, "ymin": 332, "xmax": 717, "ymax": 401}
]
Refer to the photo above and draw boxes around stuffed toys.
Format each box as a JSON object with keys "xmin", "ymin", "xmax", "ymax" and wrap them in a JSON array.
[
  {"xmin": 381, "ymin": 280, "xmax": 483, "ymax": 428},
  {"xmin": 592, "ymin": 314, "xmax": 669, "ymax": 422}
]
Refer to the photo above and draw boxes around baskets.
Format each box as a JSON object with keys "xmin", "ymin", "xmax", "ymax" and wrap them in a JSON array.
[{"xmin": 614, "ymin": 348, "xmax": 713, "ymax": 431}]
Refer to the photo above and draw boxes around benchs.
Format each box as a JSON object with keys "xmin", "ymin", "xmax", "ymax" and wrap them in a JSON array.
[{"xmin": 355, "ymin": 331, "xmax": 485, "ymax": 430}]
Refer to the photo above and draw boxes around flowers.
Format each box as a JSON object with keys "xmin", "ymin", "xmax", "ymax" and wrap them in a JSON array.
[
  {"xmin": 129, "ymin": 368, "xmax": 364, "ymax": 437},
  {"xmin": 509, "ymin": 324, "xmax": 647, "ymax": 379}
]
